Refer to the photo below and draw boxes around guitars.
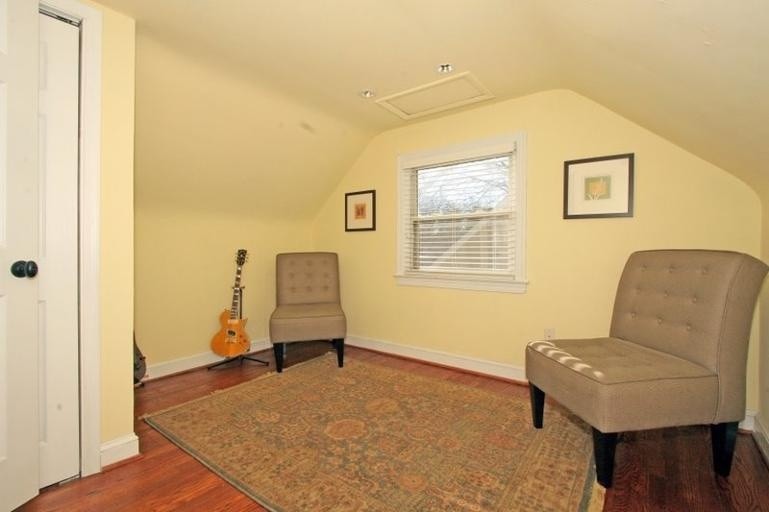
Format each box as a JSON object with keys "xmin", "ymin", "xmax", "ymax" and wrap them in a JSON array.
[{"xmin": 210, "ymin": 248, "xmax": 250, "ymax": 358}]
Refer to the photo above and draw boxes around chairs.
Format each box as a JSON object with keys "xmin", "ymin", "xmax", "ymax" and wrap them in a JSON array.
[
  {"xmin": 269, "ymin": 252, "xmax": 346, "ymax": 373},
  {"xmin": 525, "ymin": 249, "xmax": 769, "ymax": 488}
]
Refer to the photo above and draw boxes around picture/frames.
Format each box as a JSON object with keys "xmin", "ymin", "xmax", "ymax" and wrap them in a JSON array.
[
  {"xmin": 563, "ymin": 153, "xmax": 634, "ymax": 219},
  {"xmin": 344, "ymin": 190, "xmax": 376, "ymax": 231}
]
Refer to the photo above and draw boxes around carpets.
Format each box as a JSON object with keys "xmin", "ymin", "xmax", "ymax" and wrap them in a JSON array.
[{"xmin": 138, "ymin": 352, "xmax": 605, "ymax": 512}]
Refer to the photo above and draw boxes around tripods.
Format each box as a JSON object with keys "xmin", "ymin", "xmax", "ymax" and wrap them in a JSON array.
[{"xmin": 205, "ymin": 286, "xmax": 271, "ymax": 368}]
